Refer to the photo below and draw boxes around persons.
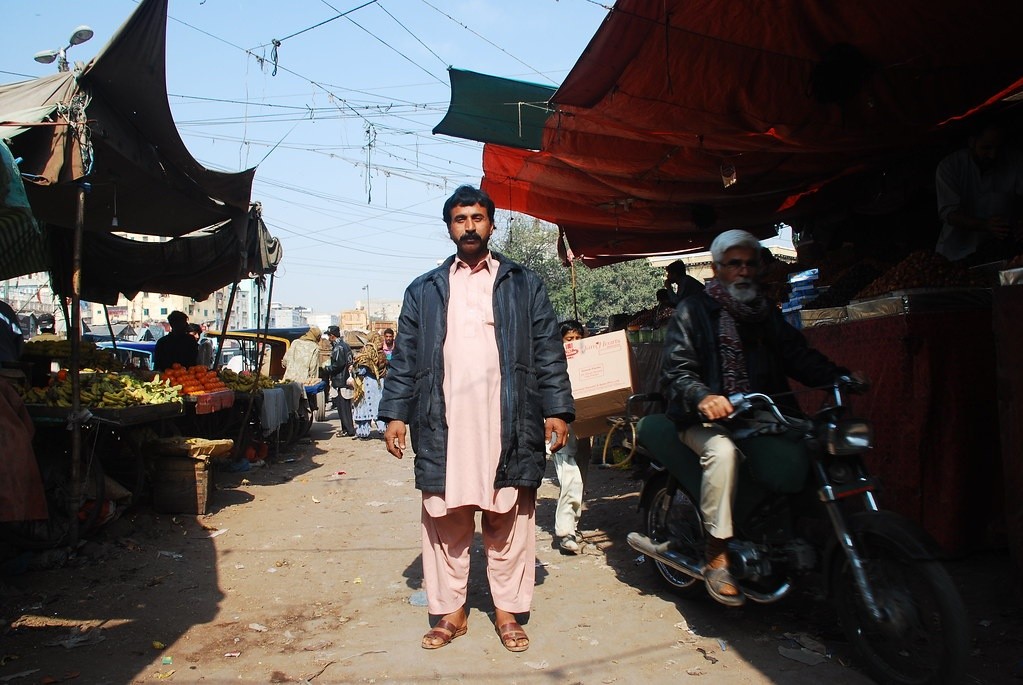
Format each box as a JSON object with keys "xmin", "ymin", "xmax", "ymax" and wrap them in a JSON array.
[
  {"xmin": 321, "ymin": 326, "xmax": 355, "ymax": 437},
  {"xmin": 378, "ymin": 186, "xmax": 576, "ymax": 653},
  {"xmin": 664, "ymin": 259, "xmax": 707, "ymax": 309},
  {"xmin": 280, "ymin": 328, "xmax": 320, "ymax": 436},
  {"xmin": 27, "ymin": 314, "xmax": 62, "ymax": 343},
  {"xmin": 661, "ymin": 228, "xmax": 872, "ymax": 605},
  {"xmin": 152, "ymin": 310, "xmax": 212, "ymax": 370},
  {"xmin": 543, "ymin": 320, "xmax": 591, "ymax": 552},
  {"xmin": 931, "ymin": 116, "xmax": 1023, "ymax": 266},
  {"xmin": 347, "ymin": 328, "xmax": 395, "ymax": 440}
]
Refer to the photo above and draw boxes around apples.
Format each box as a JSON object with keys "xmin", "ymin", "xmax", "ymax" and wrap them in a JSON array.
[
  {"xmin": 25, "ymin": 340, "xmax": 94, "ymax": 357},
  {"xmin": 150, "ymin": 363, "xmax": 228, "ymax": 395}
]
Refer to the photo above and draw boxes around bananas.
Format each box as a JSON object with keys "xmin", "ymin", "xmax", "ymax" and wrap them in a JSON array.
[
  {"xmin": 85, "ymin": 358, "xmax": 124, "ymax": 371},
  {"xmin": 222, "ymin": 368, "xmax": 275, "ymax": 391},
  {"xmin": 20, "ymin": 378, "xmax": 147, "ymax": 408}
]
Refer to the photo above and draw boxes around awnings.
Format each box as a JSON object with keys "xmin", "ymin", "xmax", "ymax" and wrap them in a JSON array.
[
  {"xmin": 430, "ymin": 1, "xmax": 1023, "ymax": 268},
  {"xmin": 0, "ymin": 0, "xmax": 282, "ymax": 305}
]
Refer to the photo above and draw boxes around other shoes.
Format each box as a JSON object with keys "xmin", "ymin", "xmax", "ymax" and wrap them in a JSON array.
[{"xmin": 336, "ymin": 431, "xmax": 349, "ymax": 437}]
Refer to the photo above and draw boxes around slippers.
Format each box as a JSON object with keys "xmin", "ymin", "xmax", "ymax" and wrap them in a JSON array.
[
  {"xmin": 421, "ymin": 620, "xmax": 468, "ymax": 649},
  {"xmin": 495, "ymin": 619, "xmax": 530, "ymax": 652}
]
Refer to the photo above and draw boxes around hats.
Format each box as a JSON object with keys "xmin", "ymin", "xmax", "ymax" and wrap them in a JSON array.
[{"xmin": 324, "ymin": 326, "xmax": 340, "ymax": 334}]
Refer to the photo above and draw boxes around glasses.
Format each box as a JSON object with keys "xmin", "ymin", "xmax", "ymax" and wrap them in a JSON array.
[{"xmin": 714, "ymin": 258, "xmax": 756, "ymax": 272}]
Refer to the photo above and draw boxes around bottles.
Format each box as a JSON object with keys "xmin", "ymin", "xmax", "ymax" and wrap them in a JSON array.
[{"xmin": 626, "ymin": 323, "xmax": 670, "ymax": 343}]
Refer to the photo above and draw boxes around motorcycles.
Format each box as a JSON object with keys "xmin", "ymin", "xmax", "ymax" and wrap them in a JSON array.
[{"xmin": 606, "ymin": 377, "xmax": 970, "ymax": 685}]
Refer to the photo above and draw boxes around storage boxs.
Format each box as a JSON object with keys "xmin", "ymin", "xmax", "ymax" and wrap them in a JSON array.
[
  {"xmin": 563, "ymin": 329, "xmax": 641, "ymax": 442},
  {"xmin": 339, "ymin": 309, "xmax": 398, "ymax": 353},
  {"xmin": 153, "ymin": 455, "xmax": 215, "ymax": 514}
]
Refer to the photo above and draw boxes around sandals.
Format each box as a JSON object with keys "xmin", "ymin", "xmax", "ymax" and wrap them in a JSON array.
[
  {"xmin": 559, "ymin": 536, "xmax": 579, "ymax": 551},
  {"xmin": 699, "ymin": 552, "xmax": 746, "ymax": 607}
]
